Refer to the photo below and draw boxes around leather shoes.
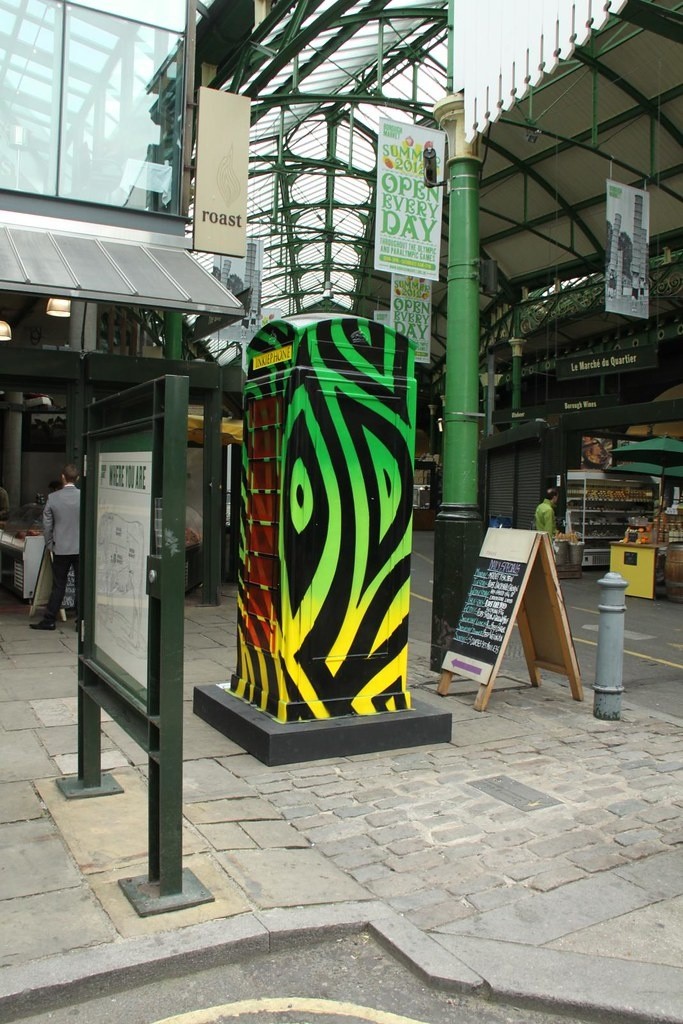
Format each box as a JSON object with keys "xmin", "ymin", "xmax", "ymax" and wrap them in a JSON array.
[{"xmin": 30, "ymin": 620, "xmax": 55, "ymax": 630}]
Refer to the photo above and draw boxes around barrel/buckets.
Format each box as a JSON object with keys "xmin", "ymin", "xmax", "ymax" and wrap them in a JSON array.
[
  {"xmin": 568, "ymin": 545, "xmax": 584, "ymax": 565},
  {"xmin": 553, "ymin": 541, "xmax": 569, "ymax": 565},
  {"xmin": 664, "ymin": 545, "xmax": 683, "ymax": 602}
]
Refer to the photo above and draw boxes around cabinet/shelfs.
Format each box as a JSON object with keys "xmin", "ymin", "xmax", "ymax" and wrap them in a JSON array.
[{"xmin": 566, "ymin": 468, "xmax": 665, "ymax": 574}]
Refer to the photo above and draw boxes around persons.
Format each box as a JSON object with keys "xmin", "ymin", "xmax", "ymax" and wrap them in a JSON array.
[
  {"xmin": 48, "ymin": 481, "xmax": 62, "ymax": 493},
  {"xmin": 0, "ymin": 487, "xmax": 10, "ymax": 521},
  {"xmin": 629, "ymin": 262, "xmax": 642, "ymax": 312},
  {"xmin": 534, "ymin": 488, "xmax": 577, "ymax": 543},
  {"xmin": 29, "ymin": 463, "xmax": 80, "ymax": 631},
  {"xmin": 606, "ymin": 267, "xmax": 625, "ymax": 300},
  {"xmin": 639, "ymin": 278, "xmax": 646, "ymax": 306}
]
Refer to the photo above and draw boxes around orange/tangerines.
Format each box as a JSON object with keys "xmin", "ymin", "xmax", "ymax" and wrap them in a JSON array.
[{"xmin": 642, "ymin": 539, "xmax": 646, "ymax": 544}]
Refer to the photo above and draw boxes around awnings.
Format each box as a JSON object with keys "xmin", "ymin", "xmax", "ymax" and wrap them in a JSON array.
[{"xmin": 0, "ymin": 226, "xmax": 246, "ymax": 317}]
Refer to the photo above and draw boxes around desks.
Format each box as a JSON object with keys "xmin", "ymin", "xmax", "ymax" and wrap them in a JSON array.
[{"xmin": 608, "ymin": 539, "xmax": 666, "ymax": 601}]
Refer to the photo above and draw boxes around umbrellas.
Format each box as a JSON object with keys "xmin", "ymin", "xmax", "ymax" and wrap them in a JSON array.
[
  {"xmin": 609, "ymin": 432, "xmax": 683, "ymax": 544},
  {"xmin": 603, "ymin": 463, "xmax": 683, "ymax": 543}
]
